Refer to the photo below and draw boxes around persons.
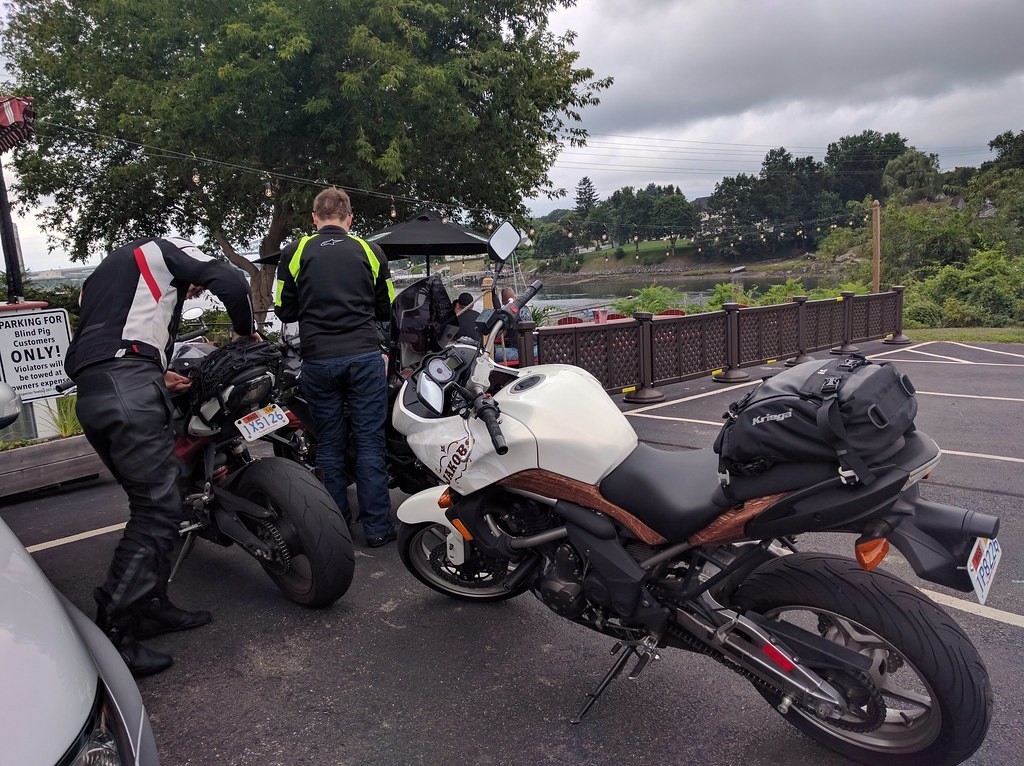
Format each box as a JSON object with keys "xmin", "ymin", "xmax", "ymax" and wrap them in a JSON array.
[
  {"xmin": 452, "ymin": 299, "xmax": 462, "ymax": 313},
  {"xmin": 458, "ymin": 293, "xmax": 482, "ymax": 345},
  {"xmin": 64, "ymin": 238, "xmax": 263, "ymax": 680},
  {"xmin": 275, "ymin": 188, "xmax": 399, "ymax": 547},
  {"xmin": 496, "ymin": 287, "xmax": 537, "ymax": 359}
]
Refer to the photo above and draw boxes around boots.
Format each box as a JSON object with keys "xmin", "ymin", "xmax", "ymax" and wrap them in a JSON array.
[
  {"xmin": 128, "ymin": 561, "xmax": 212, "ymax": 639},
  {"xmin": 91, "ymin": 586, "xmax": 174, "ymax": 674}
]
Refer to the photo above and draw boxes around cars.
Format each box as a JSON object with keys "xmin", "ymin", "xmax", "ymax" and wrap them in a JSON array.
[{"xmin": 0, "ymin": 382, "xmax": 160, "ymax": 766}]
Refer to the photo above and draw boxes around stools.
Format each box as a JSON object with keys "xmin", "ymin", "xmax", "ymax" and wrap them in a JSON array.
[
  {"xmin": 494, "ymin": 337, "xmax": 508, "ymax": 344},
  {"xmin": 498, "ymin": 358, "xmax": 538, "ymax": 366}
]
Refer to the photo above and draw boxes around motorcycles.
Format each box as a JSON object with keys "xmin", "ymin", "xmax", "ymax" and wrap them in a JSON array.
[
  {"xmin": 56, "ymin": 307, "xmax": 354, "ymax": 609},
  {"xmin": 252, "ymin": 317, "xmax": 447, "ymax": 494},
  {"xmin": 386, "ymin": 221, "xmax": 1002, "ymax": 766},
  {"xmin": 168, "ymin": 307, "xmax": 219, "ymax": 382}
]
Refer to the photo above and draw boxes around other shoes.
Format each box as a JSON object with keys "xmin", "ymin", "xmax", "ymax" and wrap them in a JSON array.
[{"xmin": 364, "ymin": 519, "xmax": 403, "ymax": 547}]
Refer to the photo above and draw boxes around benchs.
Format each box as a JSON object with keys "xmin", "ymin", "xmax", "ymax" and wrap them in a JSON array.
[{"xmin": 559, "ymin": 303, "xmax": 754, "ymax": 366}]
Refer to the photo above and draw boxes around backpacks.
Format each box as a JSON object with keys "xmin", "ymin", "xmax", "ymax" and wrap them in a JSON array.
[{"xmin": 711, "ymin": 353, "xmax": 918, "ymax": 491}]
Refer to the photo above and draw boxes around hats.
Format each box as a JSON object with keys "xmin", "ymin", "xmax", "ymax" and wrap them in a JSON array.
[{"xmin": 458, "ymin": 292, "xmax": 472, "ymax": 306}]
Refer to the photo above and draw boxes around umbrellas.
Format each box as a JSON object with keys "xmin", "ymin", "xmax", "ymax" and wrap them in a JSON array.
[
  {"xmin": 366, "ymin": 211, "xmax": 490, "ymax": 275},
  {"xmin": 251, "ymin": 250, "xmax": 408, "ymax": 266}
]
